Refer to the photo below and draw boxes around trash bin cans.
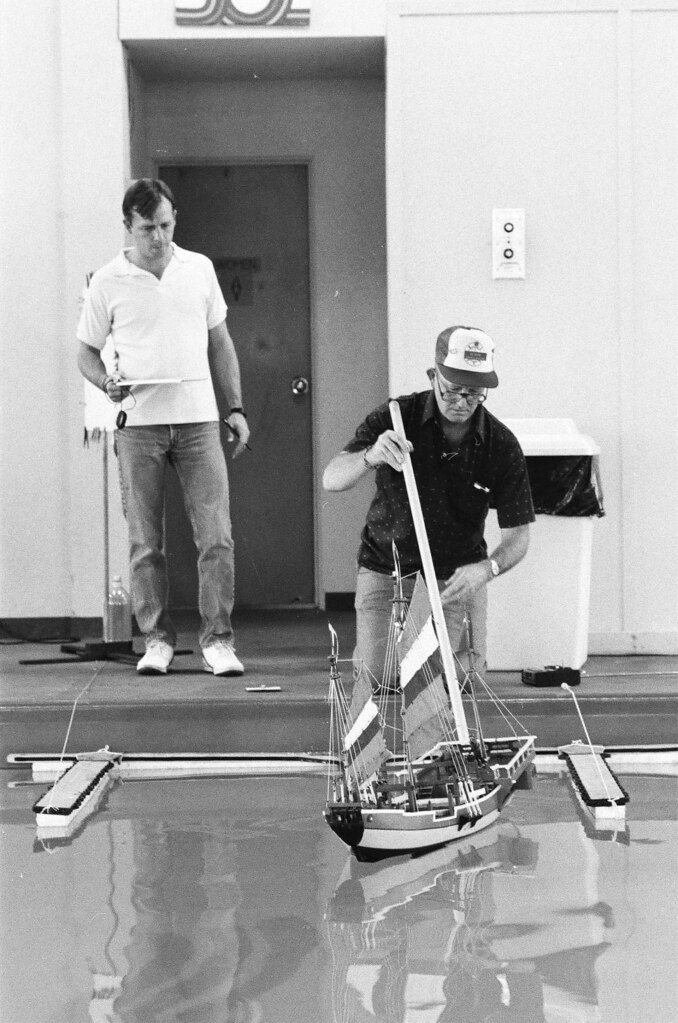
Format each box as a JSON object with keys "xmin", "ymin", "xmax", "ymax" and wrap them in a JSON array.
[{"xmin": 481, "ymin": 415, "xmax": 606, "ymax": 689}]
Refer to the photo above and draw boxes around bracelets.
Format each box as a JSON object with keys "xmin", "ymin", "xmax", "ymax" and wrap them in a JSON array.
[
  {"xmin": 363, "ymin": 445, "xmax": 380, "ymax": 470},
  {"xmin": 102, "ymin": 375, "xmax": 108, "ymax": 391},
  {"xmin": 231, "ymin": 408, "xmax": 247, "ymax": 418}
]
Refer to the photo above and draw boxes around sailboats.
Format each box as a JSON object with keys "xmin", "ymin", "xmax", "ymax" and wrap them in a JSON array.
[{"xmin": 315, "ymin": 399, "xmax": 541, "ymax": 857}]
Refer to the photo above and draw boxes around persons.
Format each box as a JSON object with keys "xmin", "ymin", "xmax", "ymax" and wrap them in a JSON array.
[
  {"xmin": 321, "ymin": 325, "xmax": 537, "ymax": 742},
  {"xmin": 75, "ymin": 177, "xmax": 250, "ymax": 676}
]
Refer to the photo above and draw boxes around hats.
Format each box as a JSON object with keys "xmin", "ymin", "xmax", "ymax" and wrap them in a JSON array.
[{"xmin": 435, "ymin": 327, "xmax": 497, "ymax": 388}]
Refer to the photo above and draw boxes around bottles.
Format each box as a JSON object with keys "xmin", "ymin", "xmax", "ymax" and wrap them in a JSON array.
[{"xmin": 104, "ymin": 577, "xmax": 134, "ymax": 647}]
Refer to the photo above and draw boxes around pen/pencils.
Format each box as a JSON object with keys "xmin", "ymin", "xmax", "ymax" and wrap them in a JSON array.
[{"xmin": 221, "ymin": 417, "xmax": 253, "ymax": 452}]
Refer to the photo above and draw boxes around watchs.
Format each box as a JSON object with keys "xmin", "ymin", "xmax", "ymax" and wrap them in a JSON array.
[{"xmin": 486, "ymin": 559, "xmax": 500, "ymax": 576}]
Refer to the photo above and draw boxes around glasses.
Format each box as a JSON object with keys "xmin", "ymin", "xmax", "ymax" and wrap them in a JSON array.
[{"xmin": 435, "ymin": 371, "xmax": 488, "ymax": 405}]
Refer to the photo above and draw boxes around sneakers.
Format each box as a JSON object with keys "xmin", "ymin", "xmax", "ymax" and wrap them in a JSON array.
[
  {"xmin": 202, "ymin": 641, "xmax": 244, "ymax": 676},
  {"xmin": 136, "ymin": 640, "xmax": 175, "ymax": 672}
]
